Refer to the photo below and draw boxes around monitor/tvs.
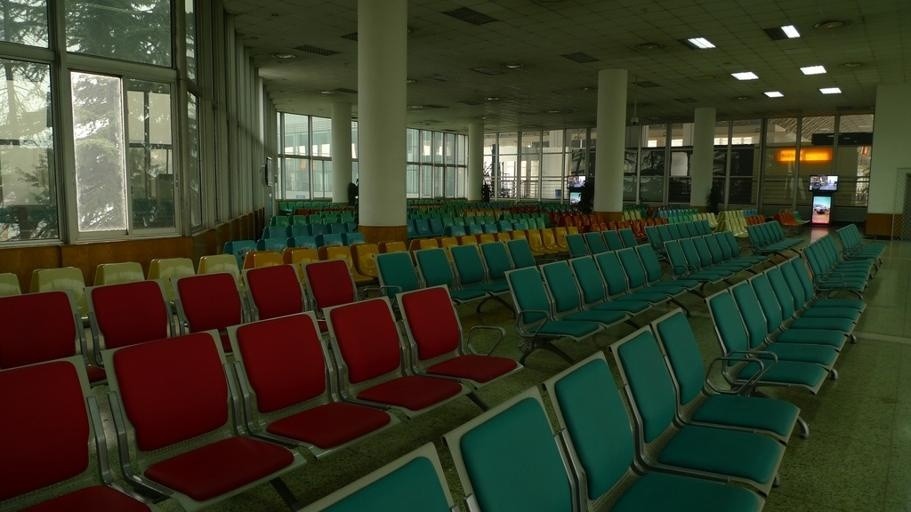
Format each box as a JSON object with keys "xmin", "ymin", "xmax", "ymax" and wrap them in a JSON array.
[
  {"xmin": 567, "ymin": 175, "xmax": 586, "ymax": 190},
  {"xmin": 808, "ymin": 175, "xmax": 839, "ymax": 192}
]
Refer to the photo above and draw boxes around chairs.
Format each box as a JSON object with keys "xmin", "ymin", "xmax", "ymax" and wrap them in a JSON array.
[
  {"xmin": 609, "ymin": 323, "xmax": 791, "ymax": 499},
  {"xmin": 542, "ymin": 347, "xmax": 768, "ymax": 511},
  {"xmin": 298, "ymin": 440, "xmax": 462, "ymax": 510},
  {"xmin": 0, "ymin": 353, "xmax": 167, "ymax": 512},
  {"xmin": 1, "ymin": 190, "xmax": 891, "ymax": 443},
  {"xmin": 224, "ymin": 308, "xmax": 403, "ymax": 464},
  {"xmin": 100, "ymin": 326, "xmax": 308, "ymax": 510},
  {"xmin": 437, "ymin": 385, "xmax": 581, "ymax": 510}
]
